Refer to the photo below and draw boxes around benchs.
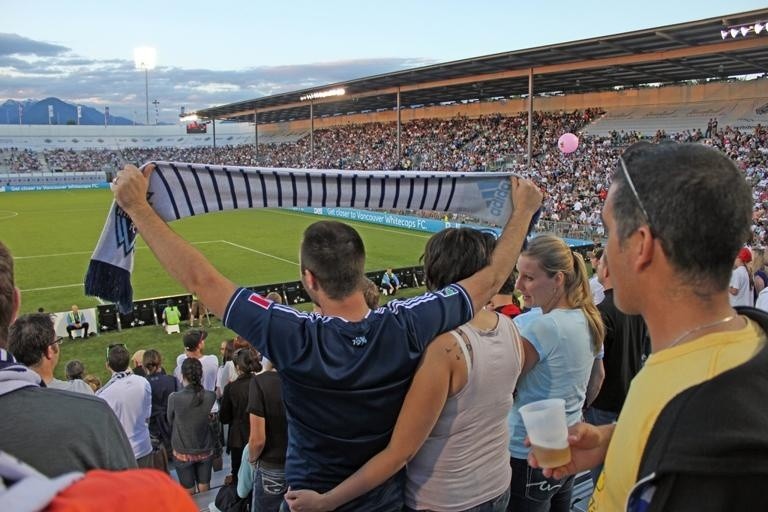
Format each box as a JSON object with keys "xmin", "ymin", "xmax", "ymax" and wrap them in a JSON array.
[
  {"xmin": 163, "ymin": 446, "xmax": 235, "ymax": 512},
  {"xmin": 578, "ymin": 101, "xmax": 767, "ymax": 140}
]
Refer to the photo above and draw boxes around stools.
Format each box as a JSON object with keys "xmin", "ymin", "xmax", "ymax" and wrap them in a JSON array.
[{"xmin": 162, "ymin": 324, "xmax": 180, "ymax": 334}]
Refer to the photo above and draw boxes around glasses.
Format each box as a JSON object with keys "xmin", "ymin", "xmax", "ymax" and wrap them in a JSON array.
[
  {"xmin": 44, "ymin": 336, "xmax": 64, "ymax": 353},
  {"xmin": 107, "ymin": 343, "xmax": 129, "ymax": 359},
  {"xmin": 617, "ymin": 140, "xmax": 684, "ymax": 240}
]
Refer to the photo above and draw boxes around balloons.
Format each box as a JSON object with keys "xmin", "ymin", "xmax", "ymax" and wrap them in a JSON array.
[{"xmin": 559, "ymin": 132, "xmax": 579, "ymax": 153}]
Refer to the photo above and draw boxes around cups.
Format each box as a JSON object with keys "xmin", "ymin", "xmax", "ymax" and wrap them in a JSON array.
[{"xmin": 518, "ymin": 397, "xmax": 573, "ymax": 468}]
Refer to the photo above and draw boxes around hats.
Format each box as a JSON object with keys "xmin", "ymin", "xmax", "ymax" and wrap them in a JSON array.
[{"xmin": 183, "ymin": 327, "xmax": 208, "ymax": 350}]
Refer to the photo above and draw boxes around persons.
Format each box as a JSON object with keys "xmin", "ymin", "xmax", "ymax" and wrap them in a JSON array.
[
  {"xmin": 521, "ymin": 141, "xmax": 767, "ymax": 509},
  {"xmin": 584, "ymin": 248, "xmax": 651, "ymax": 486},
  {"xmin": 313, "ymin": 109, "xmax": 604, "ymax": 172},
  {"xmin": 363, "ymin": 277, "xmax": 380, "ymax": 309},
  {"xmin": 509, "ymin": 234, "xmax": 606, "ymax": 510},
  {"xmin": 109, "ymin": 163, "xmax": 544, "ymax": 511},
  {"xmin": 0, "ymin": 134, "xmax": 311, "ymax": 172},
  {"xmin": 162, "ymin": 300, "xmax": 181, "ymax": 326},
  {"xmin": 506, "ymin": 130, "xmax": 702, "ymax": 245},
  {"xmin": 381, "ymin": 267, "xmax": 399, "ymax": 295},
  {"xmin": 703, "ymin": 117, "xmax": 768, "ymax": 253},
  {"xmin": 190, "ymin": 292, "xmax": 204, "ymax": 327},
  {"xmin": 283, "ymin": 228, "xmax": 526, "ymax": 510},
  {"xmin": 1, "ymin": 245, "xmax": 289, "ymax": 512},
  {"xmin": 67, "ymin": 304, "xmax": 89, "ymax": 340},
  {"xmin": 1, "ymin": 451, "xmax": 201, "ymax": 511},
  {"xmin": 729, "ymin": 248, "xmax": 767, "ymax": 311},
  {"xmin": 488, "ymin": 273, "xmax": 543, "ymax": 331}
]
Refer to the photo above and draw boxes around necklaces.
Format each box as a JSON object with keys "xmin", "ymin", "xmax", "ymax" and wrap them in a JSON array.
[{"xmin": 664, "ymin": 309, "xmax": 739, "ymax": 350}]
